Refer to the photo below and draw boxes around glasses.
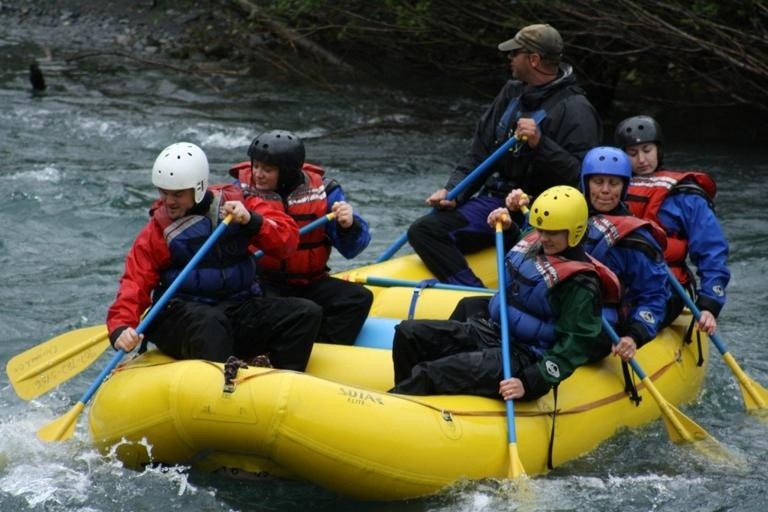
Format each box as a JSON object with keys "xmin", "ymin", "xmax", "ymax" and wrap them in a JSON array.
[{"xmin": 510, "ymin": 49, "xmax": 535, "ymax": 57}]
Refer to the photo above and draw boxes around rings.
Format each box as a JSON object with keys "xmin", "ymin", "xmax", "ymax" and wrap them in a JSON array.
[
  {"xmin": 621, "ymin": 348, "xmax": 625, "ymax": 351},
  {"xmin": 508, "ymin": 389, "xmax": 513, "ymax": 393},
  {"xmin": 624, "ymin": 352, "xmax": 629, "ymax": 354},
  {"xmin": 518, "ymin": 124, "xmax": 522, "ymax": 130},
  {"xmin": 629, "ymin": 355, "xmax": 633, "ymax": 358}
]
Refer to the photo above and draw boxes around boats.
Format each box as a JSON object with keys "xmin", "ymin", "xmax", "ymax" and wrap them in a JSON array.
[{"xmin": 89, "ymin": 246, "xmax": 710, "ymax": 501}]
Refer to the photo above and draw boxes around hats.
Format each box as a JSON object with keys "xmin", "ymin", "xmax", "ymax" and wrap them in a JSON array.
[{"xmin": 497, "ymin": 23, "xmax": 564, "ymax": 58}]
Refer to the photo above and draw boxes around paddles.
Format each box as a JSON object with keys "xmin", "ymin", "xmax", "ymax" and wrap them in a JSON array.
[
  {"xmin": 494, "ymin": 216, "xmax": 532, "ymax": 480},
  {"xmin": 6, "ymin": 200, "xmax": 340, "ymax": 400},
  {"xmin": 600, "ymin": 314, "xmax": 712, "ymax": 441},
  {"xmin": 37, "ymin": 203, "xmax": 241, "ymax": 443},
  {"xmin": 664, "ymin": 264, "xmax": 768, "ymax": 413}
]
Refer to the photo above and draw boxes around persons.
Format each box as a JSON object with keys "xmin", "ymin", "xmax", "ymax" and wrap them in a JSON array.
[
  {"xmin": 107, "ymin": 142, "xmax": 320, "ymax": 372},
  {"xmin": 613, "ymin": 115, "xmax": 731, "ymax": 335},
  {"xmin": 409, "ymin": 25, "xmax": 605, "ymax": 288},
  {"xmin": 228, "ymin": 129, "xmax": 374, "ymax": 346},
  {"xmin": 386, "ymin": 185, "xmax": 622, "ymax": 401},
  {"xmin": 449, "ymin": 147, "xmax": 671, "ymax": 367}
]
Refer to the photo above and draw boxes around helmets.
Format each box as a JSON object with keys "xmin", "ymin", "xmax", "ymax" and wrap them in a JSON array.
[
  {"xmin": 528, "ymin": 185, "xmax": 588, "ymax": 247},
  {"xmin": 580, "ymin": 146, "xmax": 631, "ymax": 199},
  {"xmin": 151, "ymin": 141, "xmax": 209, "ymax": 204},
  {"xmin": 614, "ymin": 114, "xmax": 664, "ymax": 162},
  {"xmin": 247, "ymin": 128, "xmax": 305, "ymax": 177}
]
